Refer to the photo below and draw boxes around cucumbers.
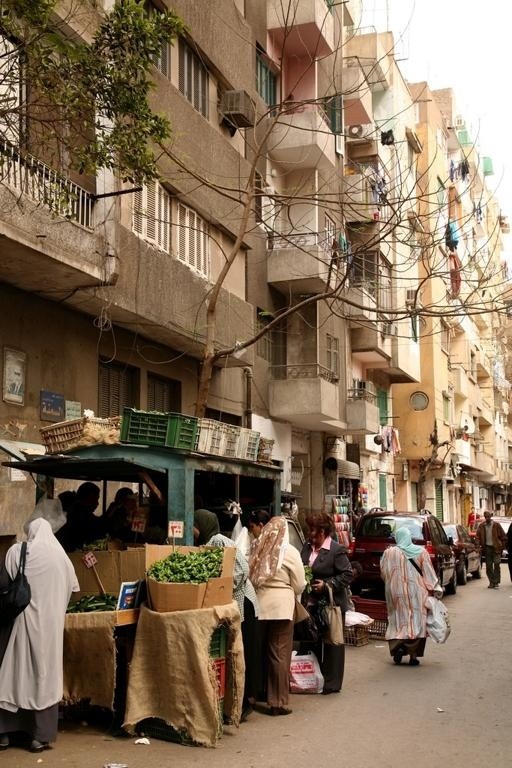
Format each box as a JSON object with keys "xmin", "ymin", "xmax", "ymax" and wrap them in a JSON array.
[{"xmin": 66, "ymin": 593, "xmax": 118, "ymax": 613}]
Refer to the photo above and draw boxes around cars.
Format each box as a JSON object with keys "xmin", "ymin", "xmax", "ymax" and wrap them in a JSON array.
[{"xmin": 346, "ymin": 509, "xmax": 512, "ymax": 599}]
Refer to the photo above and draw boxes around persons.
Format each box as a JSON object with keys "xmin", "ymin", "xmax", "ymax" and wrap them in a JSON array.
[
  {"xmin": 248, "ymin": 516, "xmax": 308, "ymax": 715},
  {"xmin": 55, "ymin": 482, "xmax": 108, "ymax": 552},
  {"xmin": 475, "ymin": 511, "xmax": 507, "ymax": 588},
  {"xmin": 0, "ymin": 518, "xmax": 80, "ymax": 751},
  {"xmin": 350, "ymin": 561, "xmax": 362, "ymax": 579},
  {"xmin": 467, "ymin": 507, "xmax": 479, "ymax": 531},
  {"xmin": 379, "ymin": 528, "xmax": 444, "ymax": 666},
  {"xmin": 300, "ymin": 512, "xmax": 353, "ymax": 695},
  {"xmin": 250, "ymin": 509, "xmax": 270, "ymax": 537},
  {"xmin": 193, "ymin": 509, "xmax": 261, "ymax": 723}
]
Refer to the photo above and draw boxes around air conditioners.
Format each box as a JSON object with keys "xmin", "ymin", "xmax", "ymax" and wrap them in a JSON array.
[
  {"xmin": 220, "ymin": 89, "xmax": 256, "ymax": 127},
  {"xmin": 358, "ymin": 381, "xmax": 374, "ymax": 398},
  {"xmin": 345, "ymin": 123, "xmax": 375, "ymax": 146}
]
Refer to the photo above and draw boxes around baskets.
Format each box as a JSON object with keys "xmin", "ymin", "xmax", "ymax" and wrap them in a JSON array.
[
  {"xmin": 208, "ymin": 622, "xmax": 224, "ymax": 658},
  {"xmin": 209, "ymin": 659, "xmax": 227, "ymax": 702},
  {"xmin": 110, "ymin": 414, "xmax": 124, "ymax": 428},
  {"xmin": 256, "ymin": 436, "xmax": 276, "ymax": 463},
  {"xmin": 195, "ymin": 417, "xmax": 260, "ymax": 463},
  {"xmin": 137, "ymin": 698, "xmax": 224, "ymax": 744},
  {"xmin": 38, "ymin": 416, "xmax": 117, "ymax": 455},
  {"xmin": 119, "ymin": 408, "xmax": 200, "ymax": 452},
  {"xmin": 368, "ymin": 617, "xmax": 388, "ymax": 640},
  {"xmin": 343, "ymin": 622, "xmax": 371, "ymax": 645}
]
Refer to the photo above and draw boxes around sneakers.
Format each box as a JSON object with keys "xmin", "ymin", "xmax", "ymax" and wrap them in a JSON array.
[
  {"xmin": 495, "ymin": 582, "xmax": 500, "ymax": 589},
  {"xmin": 1, "ymin": 737, "xmax": 10, "ymax": 750},
  {"xmin": 27, "ymin": 738, "xmax": 46, "ymax": 752},
  {"xmin": 394, "ymin": 648, "xmax": 403, "ymax": 663},
  {"xmin": 488, "ymin": 583, "xmax": 495, "ymax": 588},
  {"xmin": 267, "ymin": 703, "xmax": 292, "ymax": 715},
  {"xmin": 409, "ymin": 659, "xmax": 419, "ymax": 665}
]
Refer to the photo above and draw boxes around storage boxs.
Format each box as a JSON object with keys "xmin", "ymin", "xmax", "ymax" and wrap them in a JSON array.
[{"xmin": 66, "ymin": 541, "xmax": 237, "ymax": 613}]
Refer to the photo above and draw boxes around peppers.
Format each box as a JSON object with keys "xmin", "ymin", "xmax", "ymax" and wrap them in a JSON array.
[{"xmin": 146, "ymin": 549, "xmax": 224, "ymax": 583}]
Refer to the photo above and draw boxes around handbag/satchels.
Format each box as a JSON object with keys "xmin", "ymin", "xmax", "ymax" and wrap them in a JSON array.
[
  {"xmin": 322, "ymin": 607, "xmax": 345, "ymax": 645},
  {"xmin": 293, "ymin": 603, "xmax": 330, "ymax": 643},
  {"xmin": 1, "ymin": 572, "xmax": 33, "ymax": 621},
  {"xmin": 424, "ymin": 596, "xmax": 451, "ymax": 643}
]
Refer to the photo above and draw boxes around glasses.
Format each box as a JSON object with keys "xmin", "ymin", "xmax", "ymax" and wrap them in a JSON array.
[{"xmin": 251, "ymin": 510, "xmax": 257, "ymax": 516}]
